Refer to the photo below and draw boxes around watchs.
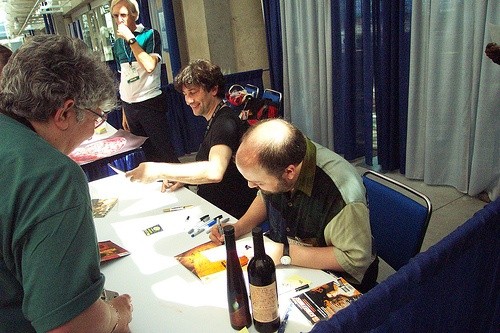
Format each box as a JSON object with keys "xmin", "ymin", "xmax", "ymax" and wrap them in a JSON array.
[
  {"xmin": 128, "ymin": 38, "xmax": 136, "ymax": 45},
  {"xmin": 280, "ymin": 243, "xmax": 292, "ymax": 266}
]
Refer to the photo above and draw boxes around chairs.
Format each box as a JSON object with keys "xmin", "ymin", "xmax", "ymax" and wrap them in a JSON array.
[
  {"xmin": 361, "ymin": 171, "xmax": 432, "ymax": 272},
  {"xmin": 229, "ymin": 84, "xmax": 282, "ymax": 118}
]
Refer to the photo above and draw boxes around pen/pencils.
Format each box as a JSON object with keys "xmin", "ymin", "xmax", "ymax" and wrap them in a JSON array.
[
  {"xmin": 157, "ymin": 180, "xmax": 176, "ymax": 182},
  {"xmin": 196, "ymin": 214, "xmax": 223, "ymax": 230}
]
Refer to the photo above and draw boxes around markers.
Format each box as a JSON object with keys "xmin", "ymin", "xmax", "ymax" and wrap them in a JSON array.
[
  {"xmin": 163, "ymin": 206, "xmax": 185, "ymax": 212},
  {"xmin": 207, "ymin": 217, "xmax": 229, "ymax": 234},
  {"xmin": 186, "ymin": 217, "xmax": 210, "ymax": 234},
  {"xmin": 190, "ymin": 221, "xmax": 216, "ymax": 237},
  {"xmin": 217, "ymin": 218, "xmax": 226, "ymax": 248}
]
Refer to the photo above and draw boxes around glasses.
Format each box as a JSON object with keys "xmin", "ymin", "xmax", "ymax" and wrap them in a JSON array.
[{"xmin": 81, "ymin": 103, "xmax": 107, "ymax": 128}]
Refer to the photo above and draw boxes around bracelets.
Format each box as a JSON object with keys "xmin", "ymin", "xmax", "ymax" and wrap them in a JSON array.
[{"xmin": 110, "ymin": 305, "xmax": 119, "ymax": 333}]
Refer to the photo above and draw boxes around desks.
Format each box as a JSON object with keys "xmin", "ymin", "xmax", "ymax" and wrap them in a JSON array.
[
  {"xmin": 66, "ymin": 119, "xmax": 146, "ymax": 185},
  {"xmin": 89, "ymin": 169, "xmax": 364, "ymax": 333}
]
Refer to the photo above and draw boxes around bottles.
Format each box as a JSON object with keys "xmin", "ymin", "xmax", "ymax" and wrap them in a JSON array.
[
  {"xmin": 223, "ymin": 225, "xmax": 252, "ymax": 330},
  {"xmin": 247, "ymin": 226, "xmax": 281, "ymax": 333}
]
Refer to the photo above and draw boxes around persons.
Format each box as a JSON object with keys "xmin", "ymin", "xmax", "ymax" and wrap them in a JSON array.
[
  {"xmin": 127, "ymin": 59, "xmax": 257, "ymax": 218},
  {"xmin": 110, "ymin": 0, "xmax": 176, "ymax": 163},
  {"xmin": 209, "ymin": 117, "xmax": 380, "ymax": 293},
  {"xmin": 0, "ymin": 34, "xmax": 133, "ymax": 333}
]
